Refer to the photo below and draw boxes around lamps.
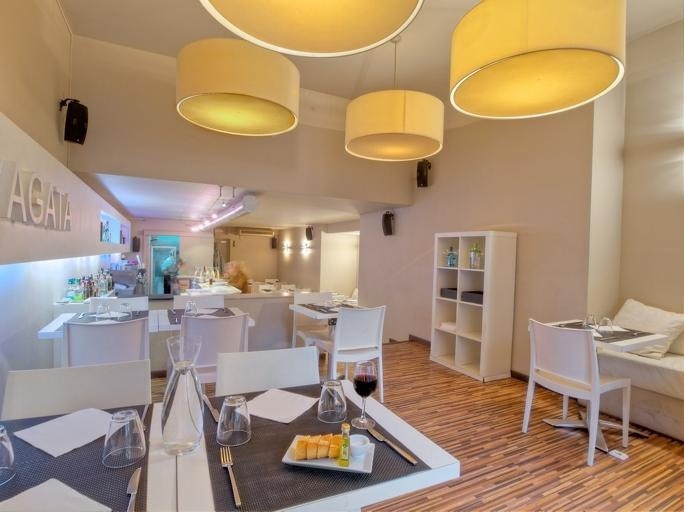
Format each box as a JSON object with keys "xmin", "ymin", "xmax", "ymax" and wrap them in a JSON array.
[
  {"xmin": 200, "ymin": 0, "xmax": 424, "ymax": 57},
  {"xmin": 173, "ymin": 39, "xmax": 300, "ymax": 137},
  {"xmin": 449, "ymin": 1, "xmax": 626, "ymax": 121},
  {"xmin": 345, "ymin": 33, "xmax": 444, "ymax": 161}
]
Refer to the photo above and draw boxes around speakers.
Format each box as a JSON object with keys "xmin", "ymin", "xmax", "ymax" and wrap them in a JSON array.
[
  {"xmin": 133, "ymin": 236, "xmax": 140, "ymax": 251},
  {"xmin": 272, "ymin": 238, "xmax": 277, "ymax": 247},
  {"xmin": 60, "ymin": 99, "xmax": 88, "ymax": 145},
  {"xmin": 306, "ymin": 226, "xmax": 313, "ymax": 240},
  {"xmin": 416, "ymin": 159, "xmax": 431, "ymax": 188},
  {"xmin": 382, "ymin": 210, "xmax": 393, "ymax": 235}
]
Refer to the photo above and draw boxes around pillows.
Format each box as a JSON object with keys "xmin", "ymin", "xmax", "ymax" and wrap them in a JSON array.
[{"xmin": 611, "ymin": 297, "xmax": 684, "ymax": 360}]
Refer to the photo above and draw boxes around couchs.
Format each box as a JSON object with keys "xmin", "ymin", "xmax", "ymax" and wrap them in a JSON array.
[{"xmin": 577, "ymin": 324, "xmax": 684, "ymax": 441}]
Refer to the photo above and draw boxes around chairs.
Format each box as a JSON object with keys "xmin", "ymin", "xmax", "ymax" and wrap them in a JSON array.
[
  {"xmin": 521, "ymin": 318, "xmax": 631, "ymax": 466},
  {"xmin": 2, "ymin": 359, "xmax": 152, "ymax": 421},
  {"xmin": 291, "ymin": 292, "xmax": 333, "ymax": 364},
  {"xmin": 316, "ymin": 305, "xmax": 385, "ymax": 403},
  {"xmin": 259, "ymin": 285, "xmax": 273, "ymax": 289},
  {"xmin": 265, "ymin": 279, "xmax": 278, "ymax": 283},
  {"xmin": 216, "ymin": 345, "xmax": 319, "ymax": 397},
  {"xmin": 173, "ymin": 294, "xmax": 223, "ymax": 308},
  {"xmin": 167, "ymin": 312, "xmax": 250, "ymax": 393},
  {"xmin": 282, "ymin": 284, "xmax": 296, "ymax": 290},
  {"xmin": 62, "ymin": 317, "xmax": 149, "ymax": 368}
]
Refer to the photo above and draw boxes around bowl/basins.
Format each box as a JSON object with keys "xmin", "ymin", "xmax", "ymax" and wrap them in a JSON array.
[{"xmin": 349, "ymin": 435, "xmax": 369, "ymax": 458}]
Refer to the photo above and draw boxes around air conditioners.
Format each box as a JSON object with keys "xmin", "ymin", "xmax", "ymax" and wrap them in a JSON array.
[{"xmin": 238, "ymin": 228, "xmax": 275, "ymax": 237}]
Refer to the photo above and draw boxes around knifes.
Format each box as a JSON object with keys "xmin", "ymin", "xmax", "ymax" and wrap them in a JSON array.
[
  {"xmin": 368, "ymin": 428, "xmax": 418, "ymax": 465},
  {"xmin": 126, "ymin": 467, "xmax": 141, "ymax": 512},
  {"xmin": 201, "ymin": 393, "xmax": 220, "ymax": 423}
]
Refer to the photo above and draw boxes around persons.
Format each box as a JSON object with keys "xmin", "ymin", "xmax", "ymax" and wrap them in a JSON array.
[{"xmin": 161, "ymin": 250, "xmax": 179, "ymax": 294}]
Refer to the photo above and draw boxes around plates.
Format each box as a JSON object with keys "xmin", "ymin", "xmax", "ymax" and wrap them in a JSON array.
[{"xmin": 282, "ymin": 435, "xmax": 375, "ymax": 474}]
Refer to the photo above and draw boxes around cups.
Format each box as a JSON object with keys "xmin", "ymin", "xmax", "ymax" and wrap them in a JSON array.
[
  {"xmin": 585, "ymin": 313, "xmax": 597, "ymax": 330},
  {"xmin": 118, "ymin": 303, "xmax": 132, "ymax": 321},
  {"xmin": 185, "ymin": 301, "xmax": 198, "ymax": 313},
  {"xmin": 351, "ymin": 362, "xmax": 378, "ymax": 430},
  {"xmin": 96, "ymin": 304, "xmax": 112, "ymax": 321},
  {"xmin": 317, "ymin": 381, "xmax": 348, "ymax": 423},
  {"xmin": 216, "ymin": 396, "xmax": 251, "ymax": 447},
  {"xmin": 102, "ymin": 409, "xmax": 146, "ymax": 468},
  {"xmin": 1, "ymin": 423, "xmax": 15, "ymax": 486},
  {"xmin": 599, "ymin": 317, "xmax": 612, "ymax": 339}
]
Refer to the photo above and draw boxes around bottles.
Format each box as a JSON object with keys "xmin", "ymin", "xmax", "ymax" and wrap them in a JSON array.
[
  {"xmin": 63, "ymin": 270, "xmax": 114, "ymax": 302},
  {"xmin": 203, "ymin": 266, "xmax": 219, "ymax": 285},
  {"xmin": 448, "ymin": 246, "xmax": 456, "ymax": 268},
  {"xmin": 338, "ymin": 424, "xmax": 350, "ymax": 466},
  {"xmin": 470, "ymin": 243, "xmax": 480, "ymax": 270}
]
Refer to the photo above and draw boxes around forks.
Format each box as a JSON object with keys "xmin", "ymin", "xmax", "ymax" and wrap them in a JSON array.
[{"xmin": 220, "ymin": 446, "xmax": 242, "ymax": 509}]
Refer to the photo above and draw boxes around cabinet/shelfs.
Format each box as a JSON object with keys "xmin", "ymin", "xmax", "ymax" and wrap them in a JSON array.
[{"xmin": 430, "ymin": 231, "xmax": 518, "ymax": 383}]
